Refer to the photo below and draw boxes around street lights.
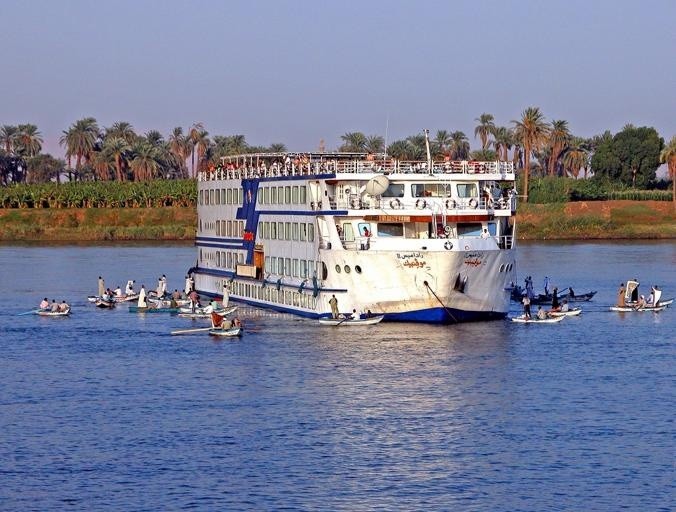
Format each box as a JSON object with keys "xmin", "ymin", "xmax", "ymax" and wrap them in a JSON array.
[{"xmin": 423, "ymin": 128, "xmax": 432, "ymax": 162}]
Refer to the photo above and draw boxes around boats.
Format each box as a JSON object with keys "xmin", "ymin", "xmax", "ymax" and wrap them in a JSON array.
[
  {"xmin": 318, "ymin": 314, "xmax": 385, "ymax": 325},
  {"xmin": 560, "ymin": 291, "xmax": 599, "ymax": 303},
  {"xmin": 177, "ymin": 305, "xmax": 238, "ymax": 317},
  {"xmin": 87, "ymin": 293, "xmax": 141, "ymax": 303},
  {"xmin": 625, "ymin": 299, "xmax": 674, "ymax": 307},
  {"xmin": 545, "ymin": 308, "xmax": 583, "ymax": 317},
  {"xmin": 96, "ymin": 299, "xmax": 112, "ymax": 307},
  {"xmin": 610, "ymin": 306, "xmax": 667, "ymax": 312},
  {"xmin": 183, "ymin": 150, "xmax": 519, "ymax": 326},
  {"xmin": 511, "ymin": 284, "xmax": 569, "ymax": 306},
  {"xmin": 208, "ymin": 326, "xmax": 242, "ymax": 336},
  {"xmin": 511, "ymin": 314, "xmax": 566, "ymax": 324},
  {"xmin": 128, "ymin": 304, "xmax": 239, "ymax": 316},
  {"xmin": 35, "ymin": 306, "xmax": 71, "ymax": 316},
  {"xmin": 147, "ymin": 289, "xmax": 192, "ymax": 308}
]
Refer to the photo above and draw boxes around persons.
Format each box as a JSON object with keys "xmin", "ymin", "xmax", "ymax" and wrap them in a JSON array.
[
  {"xmin": 201, "ymin": 150, "xmax": 489, "ymax": 176},
  {"xmin": 504, "ymin": 224, "xmax": 513, "ymax": 249},
  {"xmin": 519, "ymin": 276, "xmax": 575, "ymax": 320},
  {"xmin": 617, "ymin": 280, "xmax": 661, "ymax": 310},
  {"xmin": 438, "ymin": 226, "xmax": 445, "ymax": 238},
  {"xmin": 348, "ymin": 308, "xmax": 361, "ymax": 320},
  {"xmin": 334, "ymin": 224, "xmax": 343, "ymax": 235},
  {"xmin": 362, "ymin": 226, "xmax": 373, "ymax": 250},
  {"xmin": 482, "ymin": 182, "xmax": 519, "ymax": 209},
  {"xmin": 480, "ymin": 229, "xmax": 491, "ymax": 239},
  {"xmin": 96, "ymin": 273, "xmax": 243, "ymax": 331},
  {"xmin": 59, "ymin": 300, "xmax": 71, "ymax": 312},
  {"xmin": 329, "ymin": 294, "xmax": 340, "ymax": 319},
  {"xmin": 40, "ymin": 295, "xmax": 48, "ymax": 310},
  {"xmin": 50, "ymin": 298, "xmax": 60, "ymax": 312}
]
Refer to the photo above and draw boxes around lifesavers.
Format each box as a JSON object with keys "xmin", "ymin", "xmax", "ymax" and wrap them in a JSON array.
[
  {"xmin": 444, "ymin": 241, "xmax": 453, "ymax": 250},
  {"xmin": 469, "ymin": 198, "xmax": 479, "ymax": 209},
  {"xmin": 445, "ymin": 198, "xmax": 456, "ymax": 209},
  {"xmin": 415, "ymin": 199, "xmax": 426, "ymax": 209},
  {"xmin": 390, "ymin": 199, "xmax": 401, "ymax": 209}
]
